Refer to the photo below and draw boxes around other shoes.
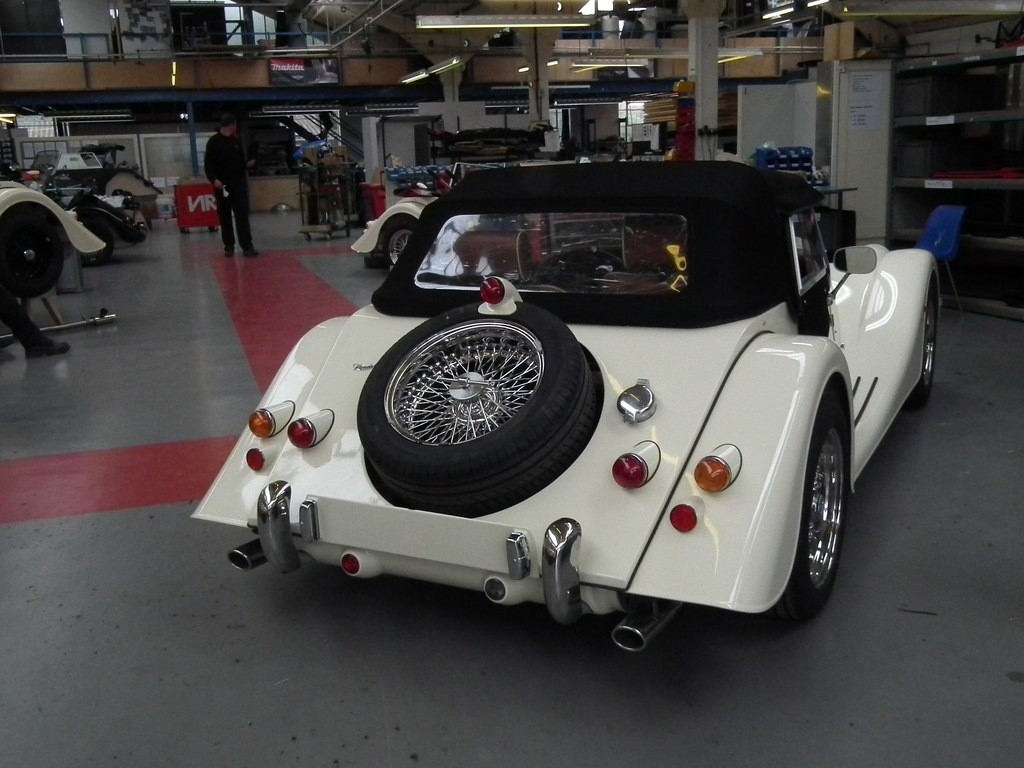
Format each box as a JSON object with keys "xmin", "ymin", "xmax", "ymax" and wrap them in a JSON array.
[
  {"xmin": 244, "ymin": 247, "xmax": 258, "ymax": 257},
  {"xmin": 25, "ymin": 339, "xmax": 70, "ymax": 357},
  {"xmin": 225, "ymin": 251, "xmax": 233, "ymax": 256}
]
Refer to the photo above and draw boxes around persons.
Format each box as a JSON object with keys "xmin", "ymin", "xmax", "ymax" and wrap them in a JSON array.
[
  {"xmin": 205, "ymin": 115, "xmax": 258, "ymax": 257},
  {"xmin": 0, "ymin": 282, "xmax": 70, "ymax": 360}
]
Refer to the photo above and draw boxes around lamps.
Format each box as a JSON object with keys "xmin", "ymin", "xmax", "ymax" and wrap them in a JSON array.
[
  {"xmin": 428, "ymin": 32, "xmax": 462, "ymax": 73},
  {"xmin": 416, "ymin": 0, "xmax": 597, "ymax": 29},
  {"xmin": 570, "ymin": 26, "xmax": 649, "ymax": 67},
  {"xmin": 400, "ymin": 68, "xmax": 429, "ymax": 83}
]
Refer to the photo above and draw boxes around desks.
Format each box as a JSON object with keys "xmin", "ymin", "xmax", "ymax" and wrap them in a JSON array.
[{"xmin": 813, "ymin": 186, "xmax": 858, "ymax": 210}]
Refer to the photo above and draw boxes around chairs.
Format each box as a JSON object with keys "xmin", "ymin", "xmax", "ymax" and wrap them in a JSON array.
[
  {"xmin": 599, "ymin": 214, "xmax": 688, "ymax": 294},
  {"xmin": 454, "ymin": 230, "xmax": 532, "ymax": 284},
  {"xmin": 914, "ymin": 205, "xmax": 967, "ymax": 319}
]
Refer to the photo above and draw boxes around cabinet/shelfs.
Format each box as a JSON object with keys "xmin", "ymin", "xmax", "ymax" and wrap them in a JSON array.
[{"xmin": 885, "ymin": 46, "xmax": 1024, "ymax": 322}]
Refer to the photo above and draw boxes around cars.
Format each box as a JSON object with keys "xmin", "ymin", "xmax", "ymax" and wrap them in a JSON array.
[
  {"xmin": 190, "ymin": 155, "xmax": 940, "ymax": 654},
  {"xmin": 350, "ymin": 161, "xmax": 504, "ymax": 266}
]
